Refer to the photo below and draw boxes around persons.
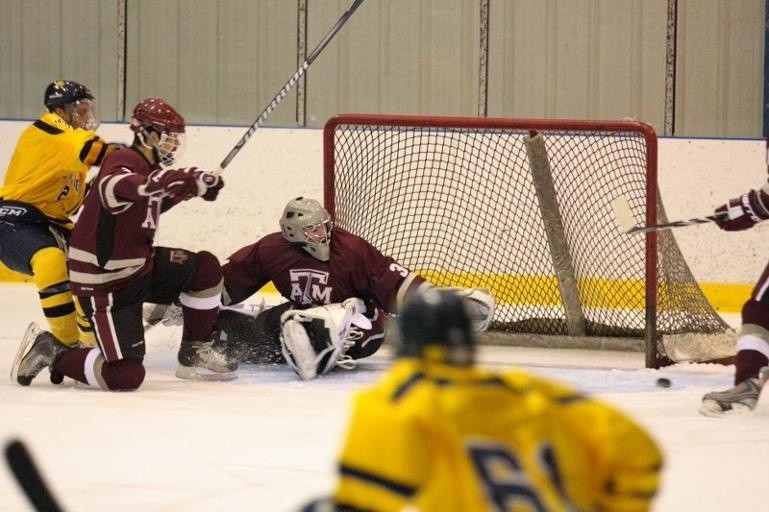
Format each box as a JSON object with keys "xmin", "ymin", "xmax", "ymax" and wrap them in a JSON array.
[
  {"xmin": 294, "ymin": 288, "xmax": 664, "ymax": 512},
  {"xmin": 143, "ymin": 196, "xmax": 495, "ymax": 381},
  {"xmin": 701, "ymin": 140, "xmax": 769, "ymax": 410},
  {"xmin": 16, "ymin": 98, "xmax": 238, "ymax": 391},
  {"xmin": 0, "ymin": 79, "xmax": 131, "ymax": 348}
]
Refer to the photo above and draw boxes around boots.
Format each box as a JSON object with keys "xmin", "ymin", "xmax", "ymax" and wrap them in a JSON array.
[
  {"xmin": 17, "ymin": 331, "xmax": 72, "ymax": 386},
  {"xmin": 702, "ymin": 366, "xmax": 768, "ymax": 409},
  {"xmin": 179, "ymin": 330, "xmax": 238, "ymax": 374}
]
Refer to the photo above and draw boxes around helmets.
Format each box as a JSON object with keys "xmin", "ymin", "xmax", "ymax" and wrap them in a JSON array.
[
  {"xmin": 393, "ymin": 286, "xmax": 474, "ymax": 360},
  {"xmin": 278, "ymin": 195, "xmax": 335, "ymax": 261},
  {"xmin": 45, "ymin": 80, "xmax": 101, "ymax": 133},
  {"xmin": 130, "ymin": 96, "xmax": 188, "ymax": 166}
]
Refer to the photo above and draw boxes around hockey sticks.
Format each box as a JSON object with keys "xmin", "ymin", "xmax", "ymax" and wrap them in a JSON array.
[
  {"xmin": 216, "ymin": 2, "xmax": 361, "ymax": 177},
  {"xmin": 612, "ymin": 197, "xmax": 720, "ymax": 234}
]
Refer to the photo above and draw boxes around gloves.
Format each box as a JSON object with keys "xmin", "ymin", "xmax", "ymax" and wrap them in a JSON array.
[{"xmin": 187, "ymin": 169, "xmax": 224, "ymax": 202}]
